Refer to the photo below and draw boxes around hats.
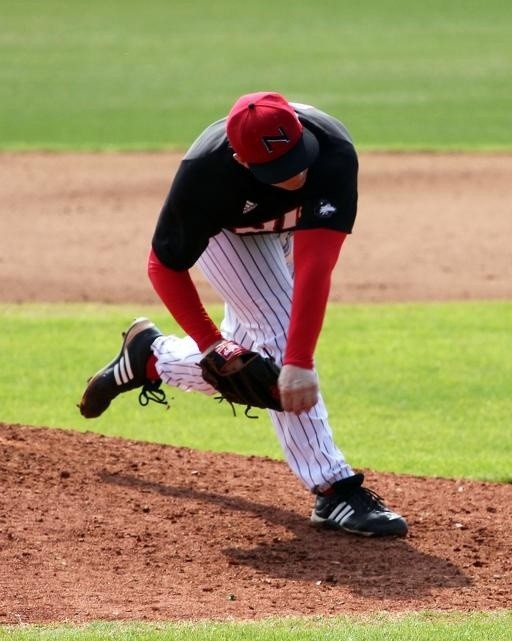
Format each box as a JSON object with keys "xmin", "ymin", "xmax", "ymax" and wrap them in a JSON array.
[{"xmin": 225, "ymin": 90, "xmax": 320, "ymax": 186}]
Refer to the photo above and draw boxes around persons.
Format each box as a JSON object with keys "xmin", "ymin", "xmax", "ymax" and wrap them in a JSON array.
[{"xmin": 80, "ymin": 90, "xmax": 410, "ymax": 537}]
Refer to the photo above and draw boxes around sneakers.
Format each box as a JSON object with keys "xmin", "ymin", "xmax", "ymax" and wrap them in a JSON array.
[
  {"xmin": 74, "ymin": 315, "xmax": 169, "ymax": 420},
  {"xmin": 348, "ymin": 506, "xmax": 351, "ymax": 512},
  {"xmin": 308, "ymin": 472, "xmax": 409, "ymax": 539}
]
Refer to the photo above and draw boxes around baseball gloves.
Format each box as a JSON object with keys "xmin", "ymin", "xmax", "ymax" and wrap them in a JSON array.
[{"xmin": 198, "ymin": 340, "xmax": 287, "ymax": 421}]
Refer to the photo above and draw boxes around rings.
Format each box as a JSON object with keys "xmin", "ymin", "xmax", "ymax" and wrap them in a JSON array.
[{"xmin": 292, "ymin": 379, "xmax": 302, "ymax": 385}]
